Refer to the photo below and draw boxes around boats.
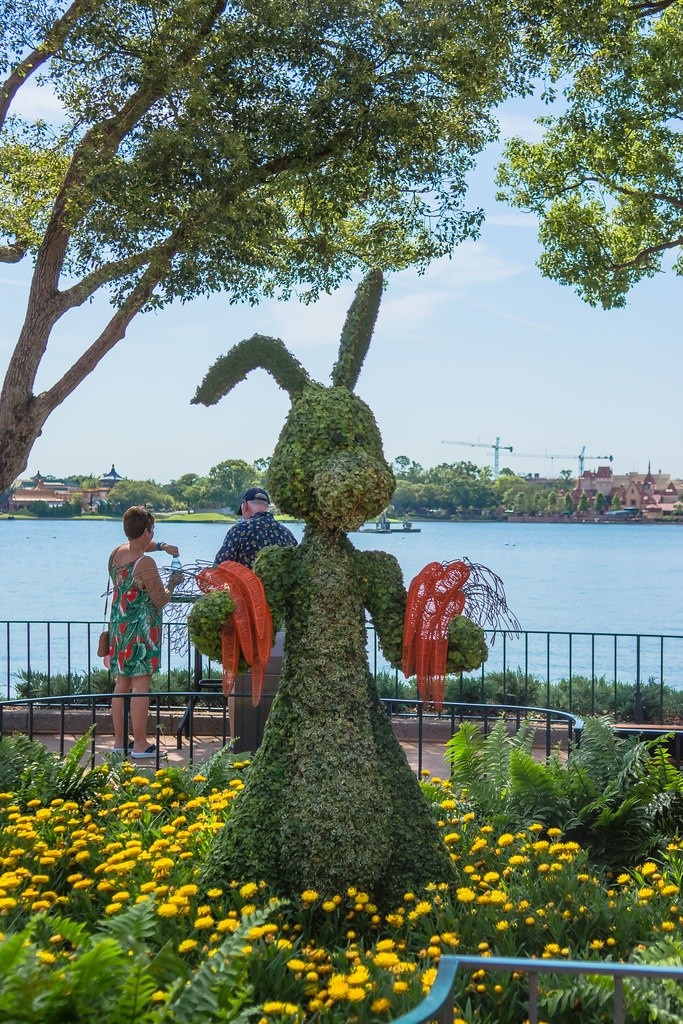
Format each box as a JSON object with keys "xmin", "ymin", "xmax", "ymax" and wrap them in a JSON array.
[{"xmin": 357, "ymin": 513, "xmax": 421, "ymax": 534}]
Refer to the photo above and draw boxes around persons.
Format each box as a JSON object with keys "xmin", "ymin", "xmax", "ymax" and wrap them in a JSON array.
[
  {"xmin": 212, "ymin": 487, "xmax": 298, "ymax": 571},
  {"xmin": 108, "ymin": 506, "xmax": 185, "ymax": 758}
]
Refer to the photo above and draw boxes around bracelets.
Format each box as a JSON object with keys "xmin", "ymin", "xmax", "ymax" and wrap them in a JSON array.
[{"xmin": 157, "ymin": 542, "xmax": 166, "ymax": 551}]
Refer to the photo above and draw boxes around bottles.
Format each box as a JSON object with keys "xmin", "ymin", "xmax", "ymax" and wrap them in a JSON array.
[{"xmin": 171, "ymin": 555, "xmax": 182, "ymax": 575}]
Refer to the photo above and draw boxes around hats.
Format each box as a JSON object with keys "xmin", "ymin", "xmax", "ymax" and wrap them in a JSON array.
[{"xmin": 236, "ymin": 486, "xmax": 272, "ymax": 515}]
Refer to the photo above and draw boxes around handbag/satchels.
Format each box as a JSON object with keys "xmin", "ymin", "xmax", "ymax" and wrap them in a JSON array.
[{"xmin": 97, "ymin": 632, "xmax": 112, "ymax": 658}]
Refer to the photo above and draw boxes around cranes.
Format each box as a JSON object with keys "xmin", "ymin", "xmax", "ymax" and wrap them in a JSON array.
[
  {"xmin": 441, "ymin": 435, "xmax": 512, "ymax": 482},
  {"xmin": 551, "ymin": 445, "xmax": 613, "ymax": 477}
]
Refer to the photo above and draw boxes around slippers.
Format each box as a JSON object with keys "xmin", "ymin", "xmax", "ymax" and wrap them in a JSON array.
[
  {"xmin": 113, "ymin": 740, "xmax": 134, "ymax": 753},
  {"xmin": 131, "ymin": 745, "xmax": 168, "ymax": 759}
]
